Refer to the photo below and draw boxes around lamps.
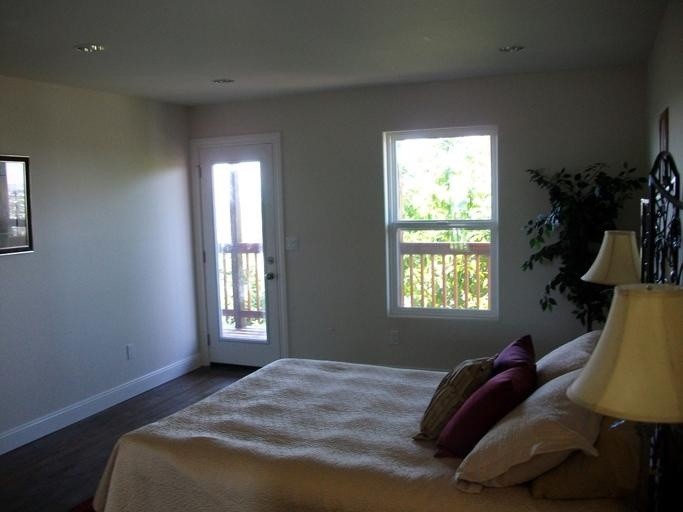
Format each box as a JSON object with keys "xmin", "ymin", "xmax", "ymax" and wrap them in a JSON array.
[
  {"xmin": 562, "ymin": 274, "xmax": 683, "ymax": 512},
  {"xmin": 573, "ymin": 222, "xmax": 645, "ymax": 294}
]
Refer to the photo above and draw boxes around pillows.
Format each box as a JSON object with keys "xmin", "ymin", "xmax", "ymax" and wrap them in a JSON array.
[
  {"xmin": 432, "ymin": 333, "xmax": 538, "ymax": 449},
  {"xmin": 535, "ymin": 329, "xmax": 602, "ymax": 388},
  {"xmin": 454, "ymin": 367, "xmax": 602, "ymax": 491},
  {"xmin": 418, "ymin": 355, "xmax": 495, "ymax": 443}
]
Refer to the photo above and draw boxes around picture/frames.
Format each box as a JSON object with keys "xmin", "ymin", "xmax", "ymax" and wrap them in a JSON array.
[{"xmin": 0, "ymin": 153, "xmax": 36, "ymax": 256}]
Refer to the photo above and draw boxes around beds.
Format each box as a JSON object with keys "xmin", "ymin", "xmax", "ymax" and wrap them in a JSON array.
[{"xmin": 85, "ymin": 147, "xmax": 683, "ymax": 512}]
